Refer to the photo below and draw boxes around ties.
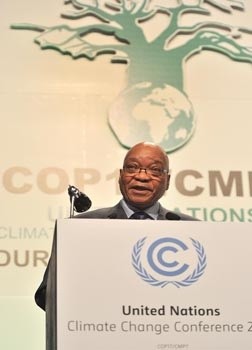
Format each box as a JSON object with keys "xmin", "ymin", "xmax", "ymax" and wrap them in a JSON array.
[{"xmin": 133, "ymin": 212, "xmax": 148, "ymax": 219}]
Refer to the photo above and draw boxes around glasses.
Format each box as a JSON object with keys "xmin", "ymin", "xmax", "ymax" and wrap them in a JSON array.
[{"xmin": 121, "ymin": 163, "xmax": 168, "ymax": 177}]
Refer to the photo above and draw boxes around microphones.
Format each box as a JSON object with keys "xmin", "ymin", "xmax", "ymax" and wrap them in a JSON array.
[{"xmin": 166, "ymin": 212, "xmax": 180, "ymax": 221}]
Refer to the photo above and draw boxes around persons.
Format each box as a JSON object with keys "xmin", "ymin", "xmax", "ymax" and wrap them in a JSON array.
[{"xmin": 34, "ymin": 140, "xmax": 204, "ymax": 313}]
